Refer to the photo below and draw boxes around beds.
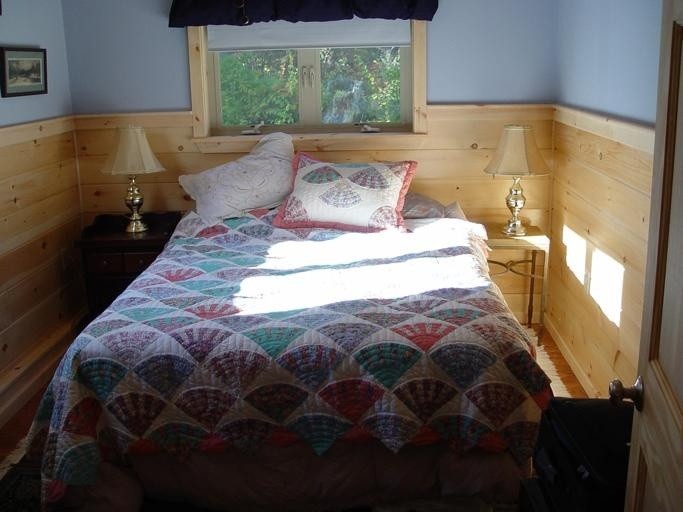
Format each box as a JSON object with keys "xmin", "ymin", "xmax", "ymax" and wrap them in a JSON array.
[{"xmin": 27, "ymin": 195, "xmax": 554, "ymax": 506}]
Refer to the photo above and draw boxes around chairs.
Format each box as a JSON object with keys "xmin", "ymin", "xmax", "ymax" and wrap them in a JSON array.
[{"xmin": 513, "ymin": 396, "xmax": 633, "ymax": 512}]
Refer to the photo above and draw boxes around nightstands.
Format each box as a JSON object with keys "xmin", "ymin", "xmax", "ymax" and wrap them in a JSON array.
[
  {"xmin": 92, "ymin": 212, "xmax": 180, "ymax": 314},
  {"xmin": 484, "ymin": 220, "xmax": 550, "ymax": 346}
]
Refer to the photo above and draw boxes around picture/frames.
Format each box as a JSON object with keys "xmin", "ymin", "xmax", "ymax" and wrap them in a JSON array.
[{"xmin": 1, "ymin": 47, "xmax": 48, "ymax": 97}]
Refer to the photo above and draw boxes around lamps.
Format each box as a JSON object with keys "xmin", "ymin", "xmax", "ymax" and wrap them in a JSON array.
[
  {"xmin": 99, "ymin": 124, "xmax": 167, "ymax": 232},
  {"xmin": 484, "ymin": 123, "xmax": 552, "ymax": 236}
]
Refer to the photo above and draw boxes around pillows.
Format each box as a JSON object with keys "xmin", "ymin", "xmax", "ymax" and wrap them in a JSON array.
[
  {"xmin": 179, "ymin": 132, "xmax": 298, "ymax": 225},
  {"xmin": 272, "ymin": 151, "xmax": 418, "ymax": 233}
]
